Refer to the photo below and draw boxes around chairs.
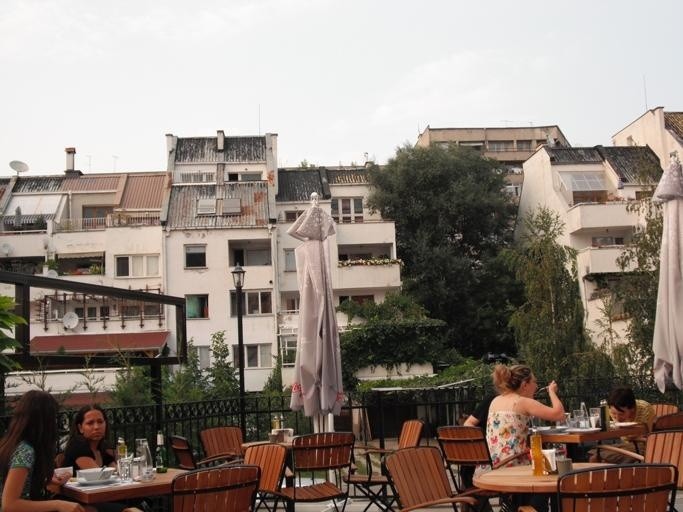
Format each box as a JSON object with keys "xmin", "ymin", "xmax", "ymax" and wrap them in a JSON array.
[
  {"xmin": 170, "ymin": 437, "xmax": 244, "ymax": 467},
  {"xmin": 202, "ymin": 428, "xmax": 272, "ymax": 511},
  {"xmin": 385, "ymin": 444, "xmax": 504, "ymax": 511},
  {"xmin": 596, "ymin": 426, "xmax": 682, "ymax": 494},
  {"xmin": 437, "ymin": 426, "xmax": 528, "ymax": 508},
  {"xmin": 172, "ymin": 465, "xmax": 259, "ymax": 511},
  {"xmin": 343, "ymin": 420, "xmax": 422, "ymax": 510},
  {"xmin": 603, "ymin": 405, "xmax": 679, "ymax": 437},
  {"xmin": 218, "ymin": 443, "xmax": 288, "ymax": 511},
  {"xmin": 521, "ymin": 464, "xmax": 681, "ymax": 512},
  {"xmin": 58, "ymin": 448, "xmax": 117, "ymax": 475},
  {"xmin": 276, "ymin": 432, "xmax": 354, "ymax": 511}
]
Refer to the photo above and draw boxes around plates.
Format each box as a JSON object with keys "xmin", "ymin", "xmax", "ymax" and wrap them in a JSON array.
[
  {"xmin": 530, "ymin": 426, "xmax": 566, "ymax": 432},
  {"xmin": 74, "ymin": 478, "xmax": 117, "ymax": 485},
  {"xmin": 615, "ymin": 421, "xmax": 637, "ymax": 429}
]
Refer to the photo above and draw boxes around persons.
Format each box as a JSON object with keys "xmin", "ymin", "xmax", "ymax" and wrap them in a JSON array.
[
  {"xmin": 588, "ymin": 387, "xmax": 657, "ymax": 464},
  {"xmin": 61, "ymin": 404, "xmax": 144, "ymax": 512},
  {"xmin": 0, "ymin": 389, "xmax": 97, "ymax": 512},
  {"xmin": 485, "ymin": 363, "xmax": 565, "ymax": 511},
  {"xmin": 459, "ymin": 393, "xmax": 499, "ymax": 512}
]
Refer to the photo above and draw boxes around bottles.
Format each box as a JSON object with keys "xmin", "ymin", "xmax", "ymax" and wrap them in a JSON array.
[
  {"xmin": 117, "ymin": 436, "xmax": 154, "ymax": 482},
  {"xmin": 154, "ymin": 431, "xmax": 167, "ymax": 473},
  {"xmin": 556, "ymin": 399, "xmax": 610, "ymax": 433},
  {"xmin": 279, "ymin": 414, "xmax": 285, "ymax": 428},
  {"xmin": 272, "ymin": 415, "xmax": 279, "ymax": 428},
  {"xmin": 530, "ymin": 433, "xmax": 544, "ymax": 476}
]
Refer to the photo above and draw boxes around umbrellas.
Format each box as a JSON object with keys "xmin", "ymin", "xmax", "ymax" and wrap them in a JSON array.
[
  {"xmin": 651, "ymin": 150, "xmax": 682, "ymax": 396},
  {"xmin": 285, "ymin": 190, "xmax": 349, "ymax": 483}
]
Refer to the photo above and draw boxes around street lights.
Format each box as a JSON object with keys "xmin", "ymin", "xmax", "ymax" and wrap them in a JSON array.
[{"xmin": 229, "ymin": 259, "xmax": 248, "ymax": 438}]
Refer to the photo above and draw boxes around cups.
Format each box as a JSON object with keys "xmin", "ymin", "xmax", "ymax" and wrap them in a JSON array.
[
  {"xmin": 268, "ymin": 433, "xmax": 278, "ymax": 442},
  {"xmin": 556, "ymin": 457, "xmax": 573, "ymax": 475}
]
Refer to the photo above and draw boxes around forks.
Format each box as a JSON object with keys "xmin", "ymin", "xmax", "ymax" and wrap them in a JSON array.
[{"xmin": 533, "ymin": 385, "xmax": 548, "ymax": 395}]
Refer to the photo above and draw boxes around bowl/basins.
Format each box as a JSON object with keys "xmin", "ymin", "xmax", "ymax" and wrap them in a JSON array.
[
  {"xmin": 272, "ymin": 429, "xmax": 291, "ymax": 442},
  {"xmin": 54, "ymin": 467, "xmax": 72, "ymax": 479},
  {"xmin": 78, "ymin": 467, "xmax": 115, "ymax": 480}
]
